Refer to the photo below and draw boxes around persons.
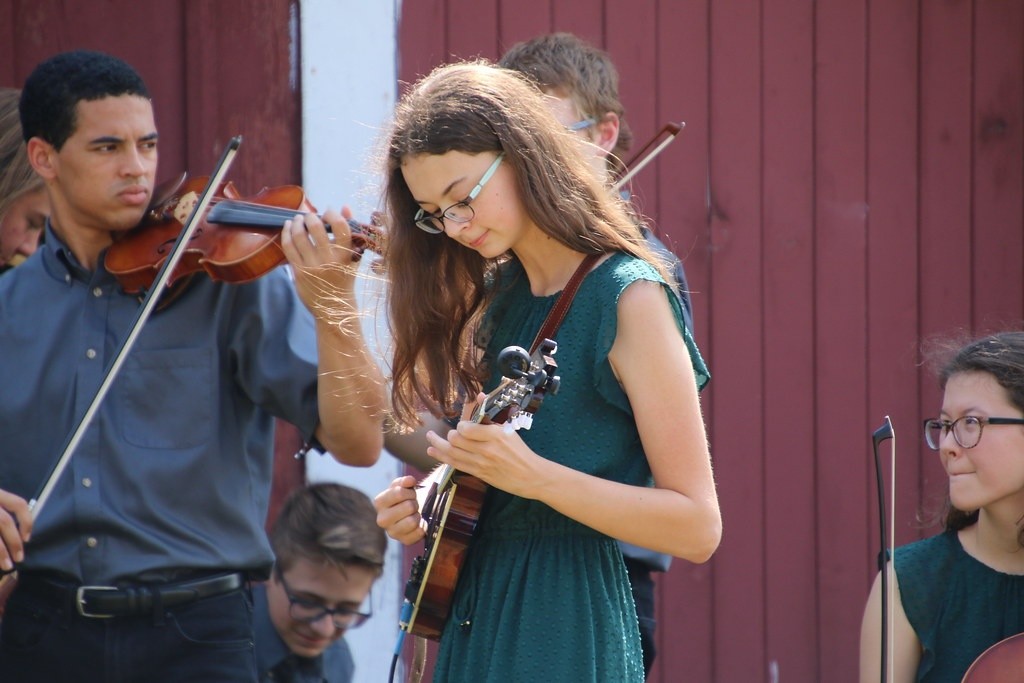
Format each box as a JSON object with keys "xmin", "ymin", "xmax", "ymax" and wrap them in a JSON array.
[
  {"xmin": 1, "ymin": 31, "xmax": 726, "ymax": 683},
  {"xmin": 856, "ymin": 330, "xmax": 1024, "ymax": 683}
]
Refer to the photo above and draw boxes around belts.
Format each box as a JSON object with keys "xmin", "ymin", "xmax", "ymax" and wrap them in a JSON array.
[{"xmin": 15, "ymin": 570, "xmax": 243, "ymax": 618}]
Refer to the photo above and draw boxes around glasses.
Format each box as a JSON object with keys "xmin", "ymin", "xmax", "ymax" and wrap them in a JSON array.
[
  {"xmin": 924, "ymin": 415, "xmax": 1024, "ymax": 450},
  {"xmin": 414, "ymin": 150, "xmax": 505, "ymax": 234},
  {"xmin": 275, "ymin": 564, "xmax": 374, "ymax": 629}
]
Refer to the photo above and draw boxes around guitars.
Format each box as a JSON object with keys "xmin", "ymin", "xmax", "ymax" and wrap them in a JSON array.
[{"xmin": 396, "ymin": 335, "xmax": 563, "ymax": 638}]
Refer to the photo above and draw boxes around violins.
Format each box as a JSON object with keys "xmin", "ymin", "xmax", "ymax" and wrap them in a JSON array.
[{"xmin": 103, "ymin": 172, "xmax": 387, "ymax": 297}]
